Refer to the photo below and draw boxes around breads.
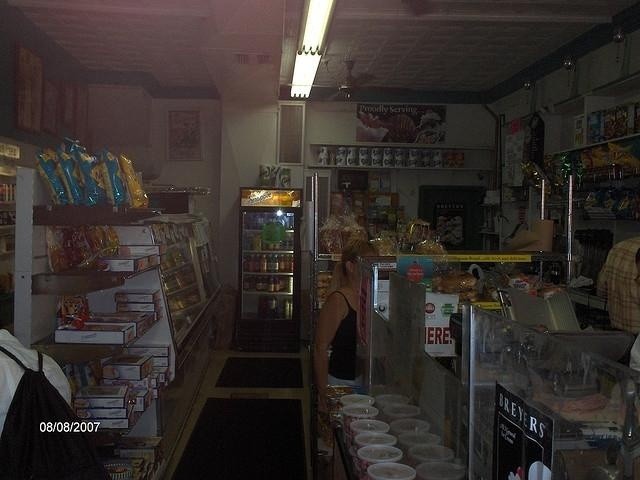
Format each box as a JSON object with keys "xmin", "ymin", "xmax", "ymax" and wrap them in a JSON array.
[
  {"xmin": 433, "ymin": 268, "xmax": 538, "ymax": 305},
  {"xmin": 308, "ymin": 270, "xmax": 334, "ymax": 313},
  {"xmin": 318, "ymin": 213, "xmax": 448, "ymax": 253}
]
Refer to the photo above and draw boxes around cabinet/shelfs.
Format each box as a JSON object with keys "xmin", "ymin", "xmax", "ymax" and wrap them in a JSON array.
[
  {"xmin": 314, "ymin": 246, "xmax": 569, "ymax": 320},
  {"xmin": 537, "ymin": 129, "xmax": 640, "ymax": 328}
]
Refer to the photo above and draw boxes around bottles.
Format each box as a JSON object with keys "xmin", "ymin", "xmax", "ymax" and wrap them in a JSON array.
[{"xmin": 243, "ymin": 211, "xmax": 293, "ymax": 291}]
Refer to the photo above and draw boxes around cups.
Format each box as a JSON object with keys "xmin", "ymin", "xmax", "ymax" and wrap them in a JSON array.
[{"xmin": 315, "ymin": 145, "xmax": 466, "ymax": 170}]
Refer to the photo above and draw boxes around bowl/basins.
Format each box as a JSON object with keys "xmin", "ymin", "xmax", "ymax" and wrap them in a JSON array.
[{"xmin": 340, "ymin": 393, "xmax": 466, "ymax": 480}]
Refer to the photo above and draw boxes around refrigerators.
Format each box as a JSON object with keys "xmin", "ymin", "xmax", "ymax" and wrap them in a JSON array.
[{"xmin": 236, "ymin": 205, "xmax": 301, "ymax": 355}]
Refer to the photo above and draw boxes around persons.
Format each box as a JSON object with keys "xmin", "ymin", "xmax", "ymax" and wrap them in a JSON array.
[
  {"xmin": 310, "ymin": 238, "xmax": 379, "ymax": 480},
  {"xmin": 629, "ymin": 246, "xmax": 640, "ymax": 374},
  {"xmin": 0, "ymin": 328, "xmax": 71, "ymax": 433},
  {"xmin": 596, "ymin": 235, "xmax": 640, "ymax": 336}
]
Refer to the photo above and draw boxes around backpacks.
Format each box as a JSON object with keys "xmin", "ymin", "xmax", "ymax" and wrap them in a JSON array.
[{"xmin": 0, "ymin": 345, "xmax": 113, "ymax": 480}]
